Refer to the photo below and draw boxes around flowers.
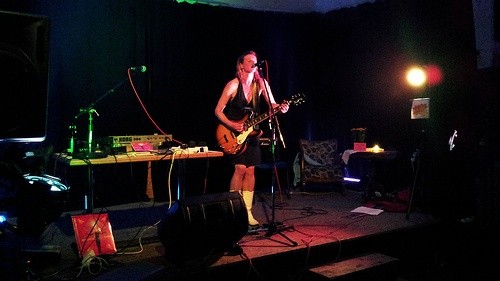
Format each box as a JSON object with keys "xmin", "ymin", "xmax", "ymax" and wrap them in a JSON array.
[{"xmin": 351, "ymin": 128, "xmax": 366, "ymax": 142}]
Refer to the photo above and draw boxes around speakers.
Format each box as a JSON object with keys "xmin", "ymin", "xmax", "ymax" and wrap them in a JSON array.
[
  {"xmin": 156, "ymin": 191, "xmax": 250, "ymax": 267},
  {"xmin": 0, "ymin": 10, "xmax": 50, "ymax": 141}
]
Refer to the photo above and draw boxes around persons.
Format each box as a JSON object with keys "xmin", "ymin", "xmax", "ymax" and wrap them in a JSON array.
[{"xmin": 215, "ymin": 51, "xmax": 290, "ymax": 225}]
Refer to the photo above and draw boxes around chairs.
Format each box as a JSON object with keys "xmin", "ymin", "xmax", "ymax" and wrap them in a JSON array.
[{"xmin": 299, "ymin": 138, "xmax": 344, "ymax": 196}]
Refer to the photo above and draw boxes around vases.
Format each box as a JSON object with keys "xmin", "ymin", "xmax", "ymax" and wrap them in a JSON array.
[{"xmin": 354, "ymin": 142, "xmax": 365, "ymax": 151}]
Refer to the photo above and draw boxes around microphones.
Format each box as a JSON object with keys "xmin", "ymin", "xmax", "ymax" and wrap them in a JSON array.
[
  {"xmin": 130, "ymin": 66, "xmax": 147, "ymax": 73},
  {"xmin": 254, "ymin": 60, "xmax": 266, "ymax": 67}
]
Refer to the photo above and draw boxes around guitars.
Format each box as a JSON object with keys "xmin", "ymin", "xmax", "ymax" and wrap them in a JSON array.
[{"xmin": 216, "ymin": 93, "xmax": 306, "ymax": 156}]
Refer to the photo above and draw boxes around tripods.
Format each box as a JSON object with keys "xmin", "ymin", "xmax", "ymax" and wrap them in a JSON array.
[{"xmin": 251, "ymin": 61, "xmax": 297, "ymax": 245}]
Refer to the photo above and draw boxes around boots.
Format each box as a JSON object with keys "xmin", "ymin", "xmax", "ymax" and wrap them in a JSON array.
[
  {"xmin": 230, "ymin": 189, "xmax": 242, "ymax": 195},
  {"xmin": 242, "ymin": 191, "xmax": 259, "ymax": 225}
]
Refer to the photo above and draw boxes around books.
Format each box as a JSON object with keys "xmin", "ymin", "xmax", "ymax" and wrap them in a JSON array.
[{"xmin": 131, "ymin": 141, "xmax": 153, "ymax": 152}]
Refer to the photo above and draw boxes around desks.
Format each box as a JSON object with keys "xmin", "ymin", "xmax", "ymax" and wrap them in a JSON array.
[
  {"xmin": 342, "ymin": 149, "xmax": 401, "ymax": 197},
  {"xmin": 52, "ymin": 148, "xmax": 223, "ymax": 214}
]
